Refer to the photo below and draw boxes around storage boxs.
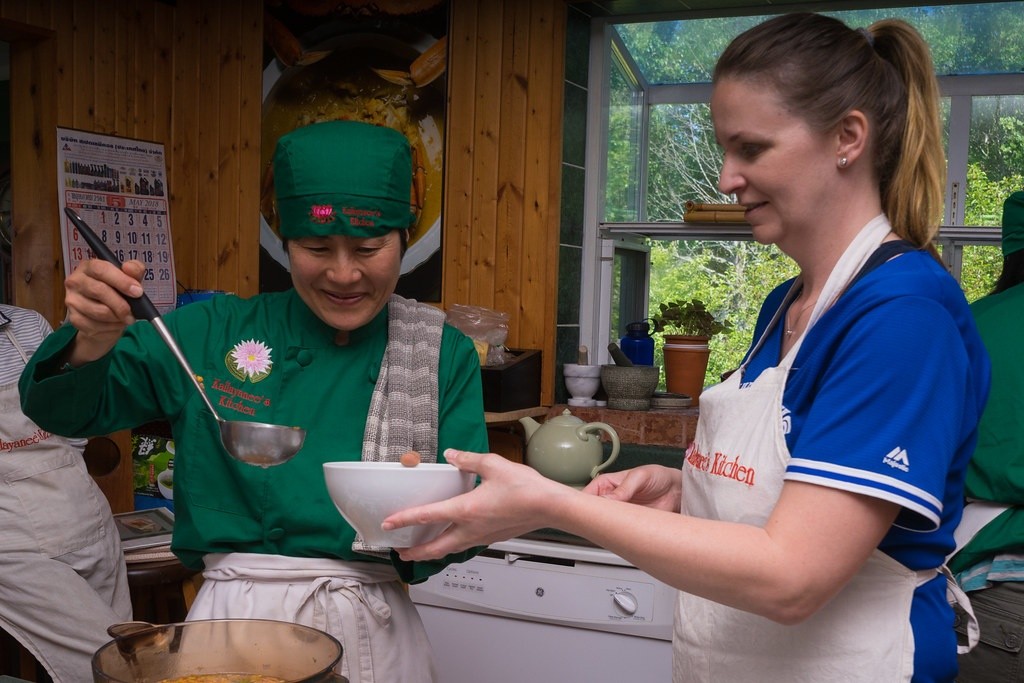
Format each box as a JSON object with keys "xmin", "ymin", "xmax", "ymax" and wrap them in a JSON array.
[{"xmin": 481, "ymin": 346, "xmax": 543, "ymax": 413}]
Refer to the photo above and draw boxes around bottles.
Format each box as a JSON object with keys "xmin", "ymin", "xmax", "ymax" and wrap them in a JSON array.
[{"xmin": 621, "ymin": 318, "xmax": 658, "ymax": 367}]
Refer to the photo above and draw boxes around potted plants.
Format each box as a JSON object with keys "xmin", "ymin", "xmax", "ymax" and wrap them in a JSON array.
[{"xmin": 654, "ymin": 297, "xmax": 733, "ymax": 344}]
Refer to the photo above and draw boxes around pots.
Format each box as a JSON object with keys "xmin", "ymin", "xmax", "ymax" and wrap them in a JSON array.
[{"xmin": 91, "ymin": 618, "xmax": 343, "ymax": 682}]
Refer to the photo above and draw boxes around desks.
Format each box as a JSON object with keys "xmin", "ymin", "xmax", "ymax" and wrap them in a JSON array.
[{"xmin": 486, "ymin": 406, "xmax": 546, "ymax": 427}]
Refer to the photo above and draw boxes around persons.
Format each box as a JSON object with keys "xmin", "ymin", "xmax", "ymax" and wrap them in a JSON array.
[
  {"xmin": 380, "ymin": 10, "xmax": 994, "ymax": 683},
  {"xmin": 945, "ymin": 190, "xmax": 1024, "ymax": 683},
  {"xmin": 18, "ymin": 118, "xmax": 489, "ymax": 683},
  {"xmin": 0, "ymin": 302, "xmax": 132, "ymax": 683}
]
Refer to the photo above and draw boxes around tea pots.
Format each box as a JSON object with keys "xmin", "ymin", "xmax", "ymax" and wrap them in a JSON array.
[{"xmin": 520, "ymin": 409, "xmax": 619, "ymax": 492}]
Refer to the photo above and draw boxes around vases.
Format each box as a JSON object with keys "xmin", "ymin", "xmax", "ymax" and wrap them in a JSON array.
[
  {"xmin": 662, "ymin": 348, "xmax": 713, "ymax": 395},
  {"xmin": 662, "ymin": 344, "xmax": 710, "ymax": 349}
]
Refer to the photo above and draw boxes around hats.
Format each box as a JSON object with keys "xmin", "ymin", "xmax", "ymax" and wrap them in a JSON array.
[
  {"xmin": 1001, "ymin": 190, "xmax": 1024, "ymax": 258},
  {"xmin": 273, "ymin": 121, "xmax": 413, "ymax": 238}
]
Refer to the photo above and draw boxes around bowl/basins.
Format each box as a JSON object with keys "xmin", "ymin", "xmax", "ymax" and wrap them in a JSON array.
[
  {"xmin": 259, "ymin": 31, "xmax": 441, "ymax": 274},
  {"xmin": 157, "ymin": 470, "xmax": 173, "ymax": 501},
  {"xmin": 324, "ymin": 463, "xmax": 476, "ymax": 547}
]
